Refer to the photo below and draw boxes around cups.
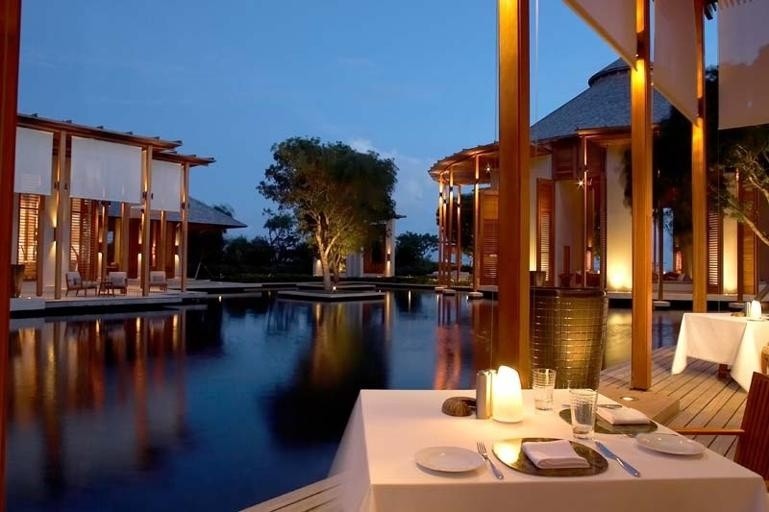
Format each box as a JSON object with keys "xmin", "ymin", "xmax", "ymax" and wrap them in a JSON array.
[
  {"xmin": 570, "ymin": 388, "xmax": 597, "ymax": 439},
  {"xmin": 532, "ymin": 365, "xmax": 555, "ymax": 410}
]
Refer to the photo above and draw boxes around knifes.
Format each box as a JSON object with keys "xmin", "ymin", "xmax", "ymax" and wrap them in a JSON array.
[{"xmin": 595, "ymin": 441, "xmax": 642, "ymax": 480}]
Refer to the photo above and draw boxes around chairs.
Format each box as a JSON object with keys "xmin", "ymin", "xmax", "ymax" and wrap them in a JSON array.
[
  {"xmin": 109, "ymin": 271, "xmax": 127, "ymax": 294},
  {"xmin": 149, "ymin": 270, "xmax": 168, "ymax": 292},
  {"xmin": 674, "ymin": 370, "xmax": 769, "ymax": 491},
  {"xmin": 65, "ymin": 270, "xmax": 81, "ymax": 296}
]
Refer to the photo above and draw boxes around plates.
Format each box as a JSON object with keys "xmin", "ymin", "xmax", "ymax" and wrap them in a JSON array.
[
  {"xmin": 414, "ymin": 447, "xmax": 484, "ymax": 473},
  {"xmin": 636, "ymin": 433, "xmax": 704, "ymax": 456}
]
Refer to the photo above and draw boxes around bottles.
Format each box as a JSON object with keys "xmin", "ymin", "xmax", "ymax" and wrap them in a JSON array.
[
  {"xmin": 475, "ymin": 368, "xmax": 496, "ymax": 419},
  {"xmin": 744, "ymin": 301, "xmax": 751, "ymax": 317}
]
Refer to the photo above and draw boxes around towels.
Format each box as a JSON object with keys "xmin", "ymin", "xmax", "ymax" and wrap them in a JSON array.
[
  {"xmin": 596, "ymin": 406, "xmax": 650, "ymax": 425},
  {"xmin": 521, "ymin": 439, "xmax": 591, "ymax": 470}
]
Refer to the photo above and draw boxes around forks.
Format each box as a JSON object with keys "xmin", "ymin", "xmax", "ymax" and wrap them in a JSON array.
[{"xmin": 477, "ymin": 440, "xmax": 504, "ymax": 480}]
